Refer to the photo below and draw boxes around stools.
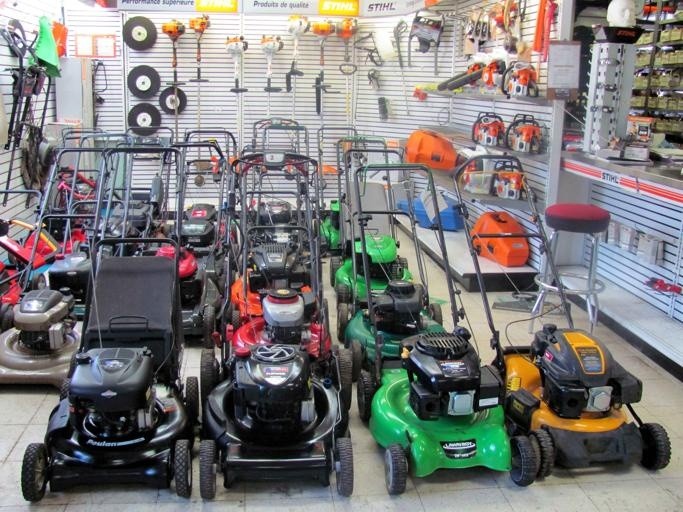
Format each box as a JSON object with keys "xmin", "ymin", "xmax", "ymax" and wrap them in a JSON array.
[{"xmin": 528, "ymin": 201, "xmax": 607, "ymax": 334}]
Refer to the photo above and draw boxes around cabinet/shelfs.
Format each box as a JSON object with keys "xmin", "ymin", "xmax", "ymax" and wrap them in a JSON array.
[
  {"xmin": 392, "ymin": 81, "xmax": 546, "ymax": 298},
  {"xmin": 631, "ymin": 1, "xmax": 683, "ymax": 123}
]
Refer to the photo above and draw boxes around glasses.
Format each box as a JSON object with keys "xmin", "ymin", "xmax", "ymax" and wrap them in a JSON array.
[
  {"xmin": 596, "ymin": 82, "xmax": 616, "ymax": 91},
  {"xmin": 598, "ymin": 58, "xmax": 620, "ymax": 66},
  {"xmin": 591, "ymin": 105, "xmax": 614, "ymax": 113}
]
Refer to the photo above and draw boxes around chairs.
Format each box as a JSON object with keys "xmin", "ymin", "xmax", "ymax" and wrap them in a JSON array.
[
  {"xmin": 345, "ymin": 183, "xmax": 390, "ymax": 243},
  {"xmin": 74, "ymin": 239, "xmax": 175, "ymax": 375}
]
